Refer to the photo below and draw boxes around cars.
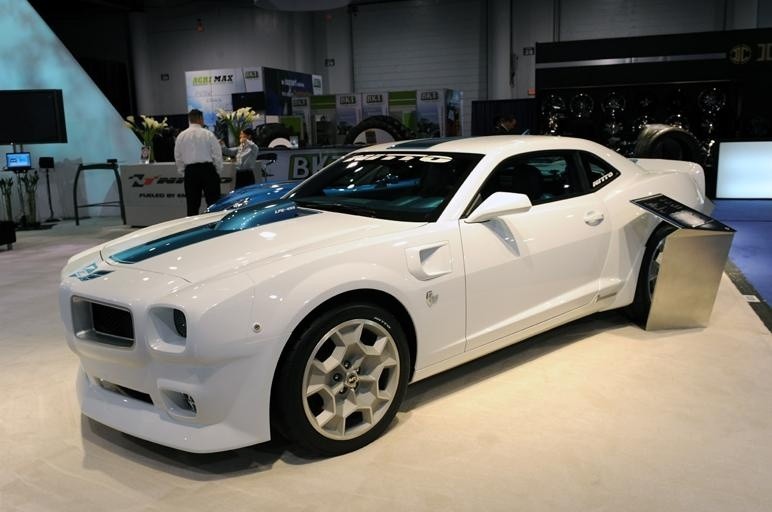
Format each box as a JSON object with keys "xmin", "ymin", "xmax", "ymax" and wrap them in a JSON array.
[{"xmin": 201, "ymin": 174, "xmax": 420, "ymax": 214}]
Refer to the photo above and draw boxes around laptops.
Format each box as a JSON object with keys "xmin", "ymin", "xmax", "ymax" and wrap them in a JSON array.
[{"xmin": 6, "ymin": 152, "xmax": 31, "ymax": 170}]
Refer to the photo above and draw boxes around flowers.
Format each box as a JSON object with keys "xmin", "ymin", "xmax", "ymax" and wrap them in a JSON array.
[
  {"xmin": 123, "ymin": 114, "xmax": 170, "ymax": 162},
  {"xmin": 216, "ymin": 106, "xmax": 261, "ymax": 146}
]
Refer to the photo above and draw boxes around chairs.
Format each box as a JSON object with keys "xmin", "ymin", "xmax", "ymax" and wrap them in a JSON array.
[{"xmin": 257, "ymin": 152, "xmax": 278, "ymax": 183}]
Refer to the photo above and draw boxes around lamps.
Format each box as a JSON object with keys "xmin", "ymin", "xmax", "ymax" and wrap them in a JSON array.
[{"xmin": 37, "ymin": 156, "xmax": 64, "ymax": 222}]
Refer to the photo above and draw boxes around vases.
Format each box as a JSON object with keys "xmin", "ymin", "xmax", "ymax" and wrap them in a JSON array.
[{"xmin": 144, "ymin": 141, "xmax": 155, "ymax": 162}]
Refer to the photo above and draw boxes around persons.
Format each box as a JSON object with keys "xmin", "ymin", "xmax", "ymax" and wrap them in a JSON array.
[
  {"xmin": 173, "ymin": 108, "xmax": 224, "ymax": 216},
  {"xmin": 217, "ymin": 128, "xmax": 259, "ymax": 191}
]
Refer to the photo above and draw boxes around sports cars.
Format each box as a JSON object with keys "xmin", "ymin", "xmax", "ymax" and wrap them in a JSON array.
[{"xmin": 58, "ymin": 134, "xmax": 707, "ymax": 459}]
